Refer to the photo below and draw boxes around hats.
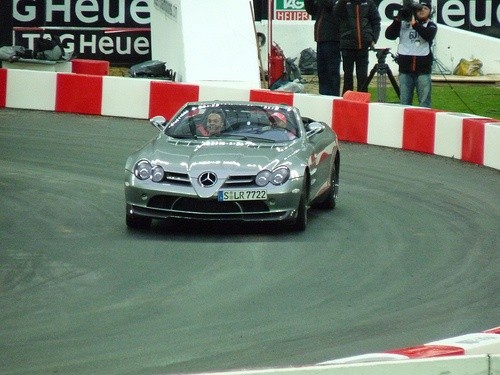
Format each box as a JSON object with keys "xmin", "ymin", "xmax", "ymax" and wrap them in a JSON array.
[
  {"xmin": 418, "ymin": 0, "xmax": 432, "ymax": 10},
  {"xmin": 268, "ymin": 112, "xmax": 287, "ymax": 122}
]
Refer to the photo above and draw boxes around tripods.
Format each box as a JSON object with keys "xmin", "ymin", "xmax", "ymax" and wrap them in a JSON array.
[{"xmin": 361, "ymin": 48, "xmax": 401, "ymax": 102}]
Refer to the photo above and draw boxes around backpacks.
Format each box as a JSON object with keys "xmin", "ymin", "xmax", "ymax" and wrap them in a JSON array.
[{"xmin": 298, "ymin": 47, "xmax": 317, "ymax": 74}]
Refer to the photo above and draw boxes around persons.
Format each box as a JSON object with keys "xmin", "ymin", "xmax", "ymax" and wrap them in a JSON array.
[
  {"xmin": 304, "ymin": 0, "xmax": 381, "ymax": 97},
  {"xmin": 385, "ymin": 1, "xmax": 437, "ymax": 107},
  {"xmin": 183, "ymin": 108, "xmax": 224, "ymax": 136},
  {"xmin": 268, "ymin": 112, "xmax": 296, "ymax": 137}
]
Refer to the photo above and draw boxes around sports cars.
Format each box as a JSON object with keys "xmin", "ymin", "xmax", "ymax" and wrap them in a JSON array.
[{"xmin": 124, "ymin": 101, "xmax": 341, "ymax": 232}]
